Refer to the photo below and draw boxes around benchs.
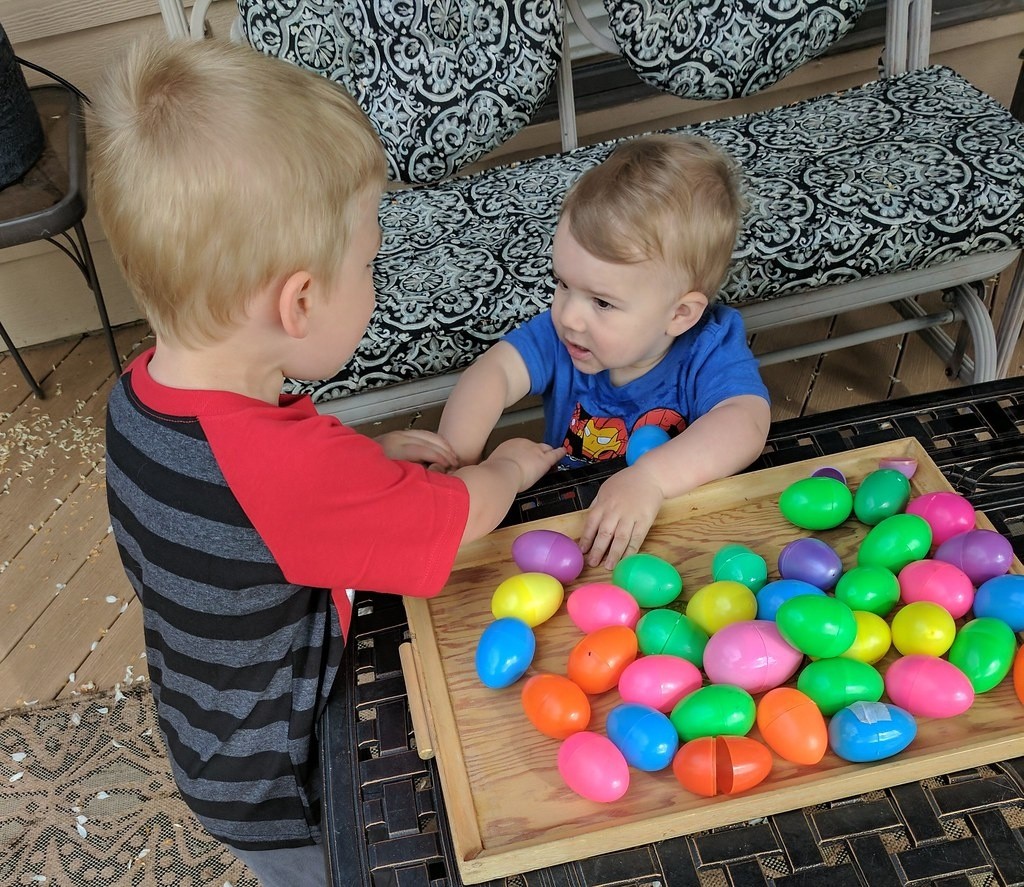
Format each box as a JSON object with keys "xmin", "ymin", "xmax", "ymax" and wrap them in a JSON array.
[{"xmin": 158, "ymin": 0, "xmax": 1023, "ymax": 431}]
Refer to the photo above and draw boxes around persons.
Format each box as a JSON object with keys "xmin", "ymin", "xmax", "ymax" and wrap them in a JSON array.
[
  {"xmin": 85, "ymin": 42, "xmax": 566, "ymax": 887},
  {"xmin": 427, "ymin": 136, "xmax": 771, "ymax": 570}
]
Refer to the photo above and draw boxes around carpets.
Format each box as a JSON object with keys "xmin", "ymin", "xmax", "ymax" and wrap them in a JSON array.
[{"xmin": 0, "ymin": 516, "xmax": 1024, "ymax": 887}]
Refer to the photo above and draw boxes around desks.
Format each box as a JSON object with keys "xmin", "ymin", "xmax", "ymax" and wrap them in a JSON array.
[
  {"xmin": 318, "ymin": 380, "xmax": 1024, "ymax": 887},
  {"xmin": 0, "ymin": 55, "xmax": 123, "ymax": 399}
]
